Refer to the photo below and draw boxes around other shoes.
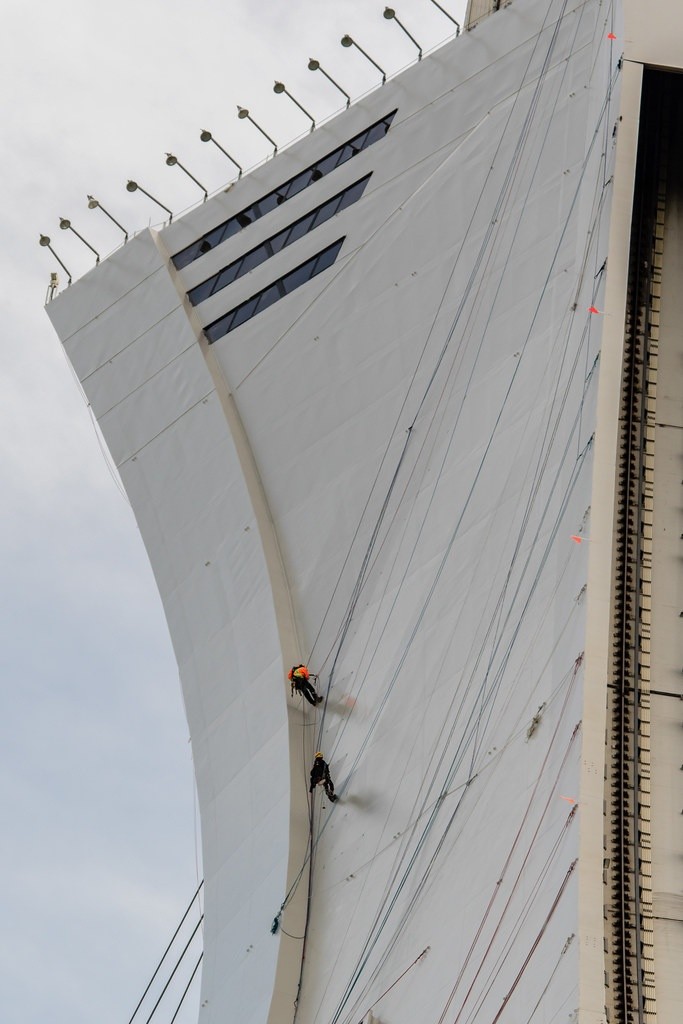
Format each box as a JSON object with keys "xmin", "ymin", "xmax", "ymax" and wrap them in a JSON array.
[
  {"xmin": 318, "ymin": 696, "xmax": 322, "ymax": 702},
  {"xmin": 313, "ymin": 702, "xmax": 316, "ymax": 706}
]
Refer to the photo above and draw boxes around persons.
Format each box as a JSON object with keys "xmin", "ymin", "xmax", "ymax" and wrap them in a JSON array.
[
  {"xmin": 311, "ymin": 752, "xmax": 337, "ymax": 802},
  {"xmin": 290, "ymin": 664, "xmax": 323, "ymax": 707}
]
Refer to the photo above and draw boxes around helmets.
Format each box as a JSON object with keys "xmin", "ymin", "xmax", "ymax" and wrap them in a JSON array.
[{"xmin": 314, "ymin": 751, "xmax": 324, "ymax": 757}]
